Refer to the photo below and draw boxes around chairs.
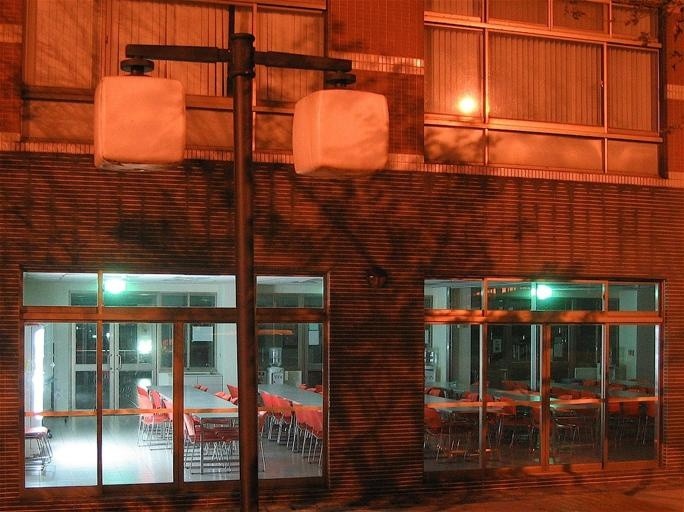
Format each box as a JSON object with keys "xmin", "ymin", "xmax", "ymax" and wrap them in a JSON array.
[
  {"xmin": 424, "ymin": 378, "xmax": 657, "ymax": 472},
  {"xmin": 137, "ymin": 382, "xmax": 324, "ymax": 476},
  {"xmin": 24, "ymin": 426, "xmax": 53, "ymax": 472}
]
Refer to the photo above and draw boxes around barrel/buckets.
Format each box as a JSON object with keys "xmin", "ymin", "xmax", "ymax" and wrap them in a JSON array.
[{"xmin": 268, "ymin": 347, "xmax": 282, "ymax": 367}]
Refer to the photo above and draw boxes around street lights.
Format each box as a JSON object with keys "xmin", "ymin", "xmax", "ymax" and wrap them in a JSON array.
[{"xmin": 94, "ymin": 32, "xmax": 389, "ymax": 512}]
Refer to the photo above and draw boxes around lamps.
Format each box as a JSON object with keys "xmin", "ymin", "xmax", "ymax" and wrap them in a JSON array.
[{"xmin": 457, "ymin": 95, "xmax": 476, "ymax": 121}]
